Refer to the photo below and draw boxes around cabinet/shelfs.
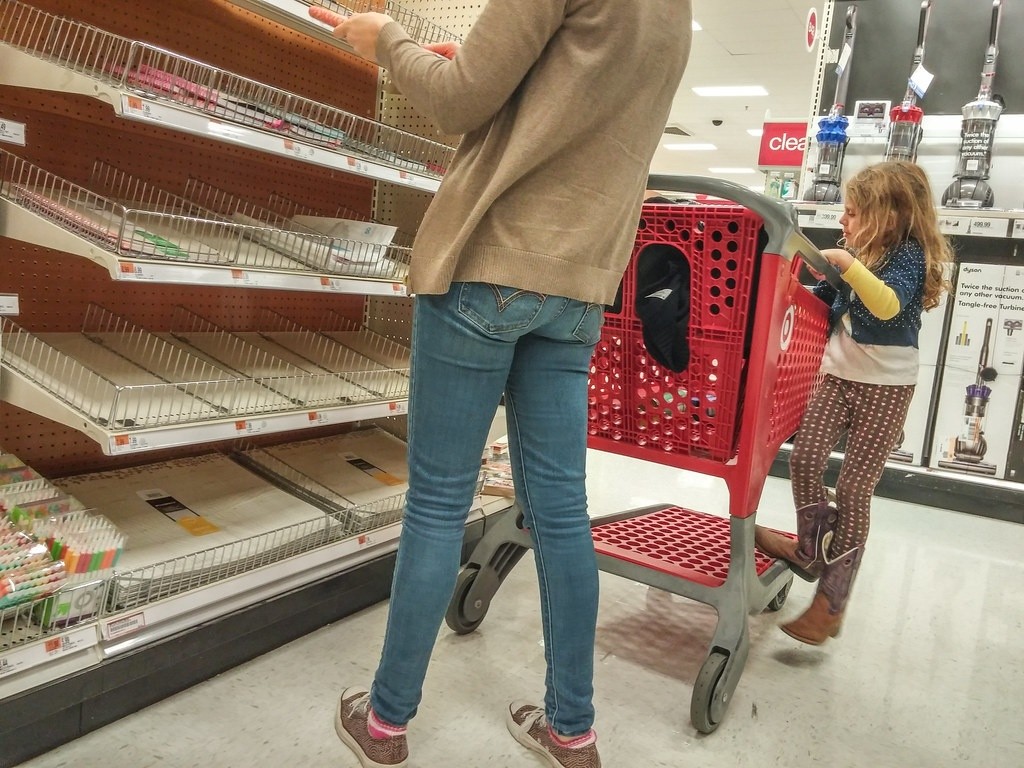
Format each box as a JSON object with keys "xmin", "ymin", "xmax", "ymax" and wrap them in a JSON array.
[
  {"xmin": 0, "ymin": 0, "xmax": 517, "ymax": 768},
  {"xmin": 760, "ymin": 0, "xmax": 1023, "ymax": 526},
  {"xmin": 763, "ymin": 169, "xmax": 801, "ymax": 201}
]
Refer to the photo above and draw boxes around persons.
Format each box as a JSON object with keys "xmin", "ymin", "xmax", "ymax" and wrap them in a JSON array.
[
  {"xmin": 332, "ymin": 0, "xmax": 692, "ymax": 768},
  {"xmin": 755, "ymin": 161, "xmax": 958, "ymax": 645}
]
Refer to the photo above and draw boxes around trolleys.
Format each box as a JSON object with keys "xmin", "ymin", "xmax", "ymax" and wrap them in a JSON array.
[{"xmin": 443, "ymin": 174, "xmax": 851, "ymax": 735}]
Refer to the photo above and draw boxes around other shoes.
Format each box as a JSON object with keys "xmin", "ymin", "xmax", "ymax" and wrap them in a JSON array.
[
  {"xmin": 753, "ymin": 525, "xmax": 818, "ymax": 582},
  {"xmin": 777, "ymin": 591, "xmax": 844, "ymax": 644}
]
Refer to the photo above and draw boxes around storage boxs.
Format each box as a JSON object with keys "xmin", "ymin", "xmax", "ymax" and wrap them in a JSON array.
[{"xmin": 885, "ymin": 251, "xmax": 1024, "ymax": 482}]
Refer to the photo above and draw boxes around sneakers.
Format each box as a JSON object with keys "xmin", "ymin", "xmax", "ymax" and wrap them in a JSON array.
[
  {"xmin": 505, "ymin": 698, "xmax": 601, "ymax": 768},
  {"xmin": 333, "ymin": 683, "xmax": 408, "ymax": 768}
]
